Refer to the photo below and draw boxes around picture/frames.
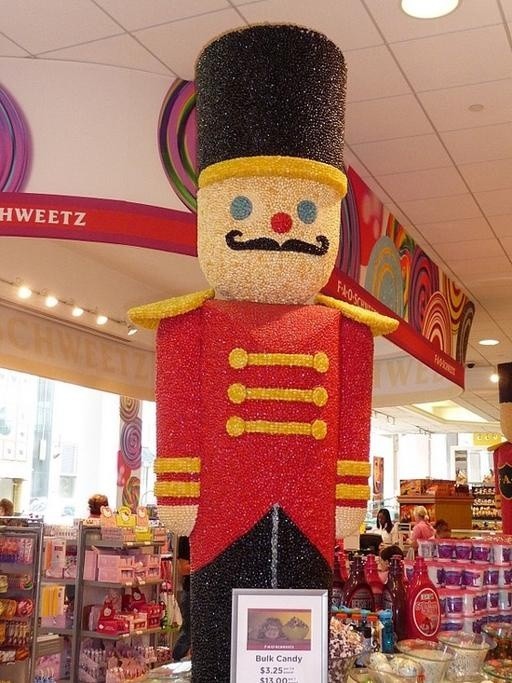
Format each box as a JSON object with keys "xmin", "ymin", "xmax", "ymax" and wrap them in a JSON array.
[{"xmin": 230, "ymin": 587, "xmax": 330, "ymax": 681}]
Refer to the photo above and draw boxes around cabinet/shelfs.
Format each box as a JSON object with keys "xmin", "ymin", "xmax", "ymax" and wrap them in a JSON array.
[
  {"xmin": 371, "ymin": 478, "xmax": 512, "ymax": 549},
  {"xmin": 61, "ymin": 513, "xmax": 184, "ymax": 682},
  {"xmin": 0, "ymin": 515, "xmax": 62, "ymax": 682}
]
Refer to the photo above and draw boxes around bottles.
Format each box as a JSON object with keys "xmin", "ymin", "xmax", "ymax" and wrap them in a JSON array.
[
  {"xmin": 417, "ymin": 536, "xmax": 512, "ymax": 635},
  {"xmin": 330, "ymin": 552, "xmax": 441, "ymax": 643},
  {"xmin": 330, "ymin": 599, "xmax": 395, "ymax": 664}
]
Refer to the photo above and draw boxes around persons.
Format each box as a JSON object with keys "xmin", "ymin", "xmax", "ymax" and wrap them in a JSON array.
[
  {"xmin": 163, "ymin": 535, "xmax": 195, "ymax": 663},
  {"xmin": 429, "ymin": 519, "xmax": 450, "ymax": 541},
  {"xmin": 0, "ymin": 496, "xmax": 13, "ymax": 523},
  {"xmin": 412, "ymin": 506, "xmax": 436, "ymax": 552},
  {"xmin": 86, "ymin": 493, "xmax": 108, "ymax": 518},
  {"xmin": 371, "ymin": 508, "xmax": 393, "ymax": 536}
]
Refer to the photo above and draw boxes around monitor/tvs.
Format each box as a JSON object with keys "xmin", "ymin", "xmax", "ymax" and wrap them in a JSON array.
[{"xmin": 359, "ymin": 534, "xmax": 384, "ymax": 553}]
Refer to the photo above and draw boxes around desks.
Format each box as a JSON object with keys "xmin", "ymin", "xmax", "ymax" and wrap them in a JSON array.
[{"xmin": 124, "ymin": 651, "xmax": 509, "ymax": 682}]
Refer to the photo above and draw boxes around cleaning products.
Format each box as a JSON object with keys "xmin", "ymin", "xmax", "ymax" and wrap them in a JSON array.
[{"xmin": 333, "ymin": 544, "xmax": 443, "ymax": 668}]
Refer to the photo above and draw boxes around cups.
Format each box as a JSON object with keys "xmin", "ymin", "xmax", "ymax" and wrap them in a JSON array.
[{"xmin": 365, "ymin": 622, "xmax": 512, "ymax": 683}]
